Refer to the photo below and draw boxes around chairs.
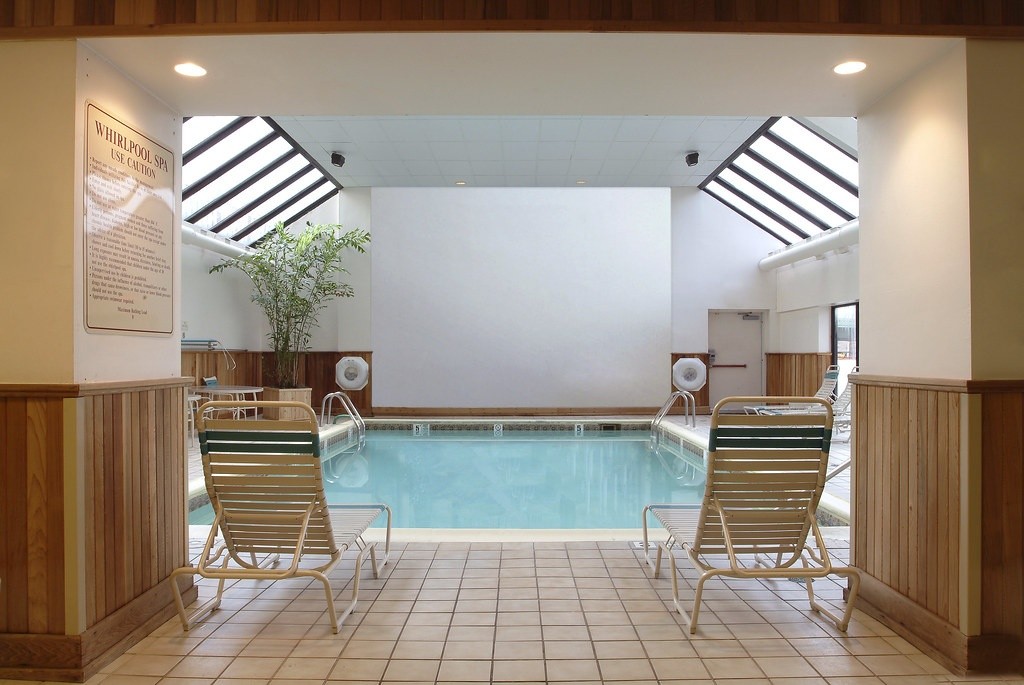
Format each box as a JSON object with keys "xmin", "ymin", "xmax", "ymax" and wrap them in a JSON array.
[
  {"xmin": 169, "ymin": 398, "xmax": 393, "ymax": 634},
  {"xmin": 188, "ymin": 375, "xmax": 246, "ymax": 446},
  {"xmin": 742, "ymin": 363, "xmax": 859, "ymax": 443},
  {"xmin": 642, "ymin": 395, "xmax": 860, "ymax": 631}
]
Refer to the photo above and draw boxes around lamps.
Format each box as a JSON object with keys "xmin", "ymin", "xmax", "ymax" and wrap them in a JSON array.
[
  {"xmin": 685, "ymin": 152, "xmax": 700, "ymax": 166},
  {"xmin": 331, "ymin": 153, "xmax": 344, "ymax": 167}
]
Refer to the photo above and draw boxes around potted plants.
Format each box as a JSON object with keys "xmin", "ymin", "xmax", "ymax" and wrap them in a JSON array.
[{"xmin": 209, "ymin": 220, "xmax": 372, "ymax": 420}]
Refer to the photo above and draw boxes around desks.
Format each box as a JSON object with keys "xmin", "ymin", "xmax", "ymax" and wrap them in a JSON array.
[{"xmin": 187, "ymin": 385, "xmax": 264, "ymax": 420}]
